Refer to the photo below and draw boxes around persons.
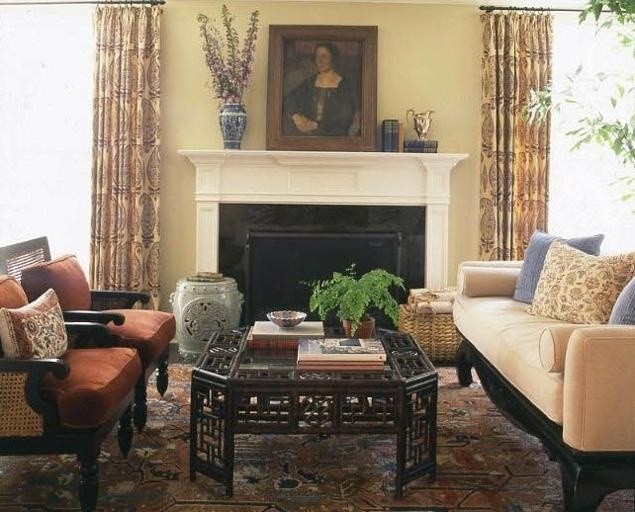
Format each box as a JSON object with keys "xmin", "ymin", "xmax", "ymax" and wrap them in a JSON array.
[{"xmin": 284, "ymin": 42, "xmax": 358, "ymax": 136}]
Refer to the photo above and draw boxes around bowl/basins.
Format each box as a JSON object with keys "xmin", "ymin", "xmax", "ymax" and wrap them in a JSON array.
[{"xmin": 266, "ymin": 310, "xmax": 307, "ymax": 327}]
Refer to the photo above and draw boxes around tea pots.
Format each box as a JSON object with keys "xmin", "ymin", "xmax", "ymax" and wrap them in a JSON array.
[{"xmin": 404, "ymin": 108, "xmax": 438, "ymax": 141}]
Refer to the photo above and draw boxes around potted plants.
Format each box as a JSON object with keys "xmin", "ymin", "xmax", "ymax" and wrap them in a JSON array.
[{"xmin": 301, "ymin": 266, "xmax": 409, "ymax": 337}]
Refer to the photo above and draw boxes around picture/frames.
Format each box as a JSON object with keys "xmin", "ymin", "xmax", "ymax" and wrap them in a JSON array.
[{"xmin": 265, "ymin": 23, "xmax": 380, "ymax": 154}]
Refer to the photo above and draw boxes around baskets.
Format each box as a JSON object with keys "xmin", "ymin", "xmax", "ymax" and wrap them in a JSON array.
[{"xmin": 399, "ymin": 288, "xmax": 464, "ymax": 361}]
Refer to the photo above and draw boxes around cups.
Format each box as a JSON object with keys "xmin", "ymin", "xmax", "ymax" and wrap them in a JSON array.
[{"xmin": 382, "ymin": 118, "xmax": 401, "ymax": 153}]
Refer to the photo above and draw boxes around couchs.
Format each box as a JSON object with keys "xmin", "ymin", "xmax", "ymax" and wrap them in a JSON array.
[{"xmin": 446, "ymin": 227, "xmax": 635, "ymax": 511}]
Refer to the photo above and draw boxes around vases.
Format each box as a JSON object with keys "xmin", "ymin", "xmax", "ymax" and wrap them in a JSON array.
[{"xmin": 217, "ymin": 106, "xmax": 245, "ymax": 150}]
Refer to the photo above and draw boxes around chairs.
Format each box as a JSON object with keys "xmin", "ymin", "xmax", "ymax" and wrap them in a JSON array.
[
  {"xmin": 0, "ymin": 235, "xmax": 175, "ymax": 433},
  {"xmin": 0, "ymin": 271, "xmax": 146, "ymax": 512}
]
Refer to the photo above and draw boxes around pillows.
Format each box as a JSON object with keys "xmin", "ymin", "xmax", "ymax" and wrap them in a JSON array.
[
  {"xmin": 512, "ymin": 231, "xmax": 603, "ymax": 310},
  {"xmin": 0, "ymin": 288, "xmax": 68, "ymax": 366},
  {"xmin": 608, "ymin": 278, "xmax": 634, "ymax": 323},
  {"xmin": 24, "ymin": 256, "xmax": 93, "ymax": 313},
  {"xmin": 527, "ymin": 243, "xmax": 634, "ymax": 325}
]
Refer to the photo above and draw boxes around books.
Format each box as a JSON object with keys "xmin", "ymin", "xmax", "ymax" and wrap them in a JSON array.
[{"xmin": 296, "ymin": 338, "xmax": 387, "ymax": 372}]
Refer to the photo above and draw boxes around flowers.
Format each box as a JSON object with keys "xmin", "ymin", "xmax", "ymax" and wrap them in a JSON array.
[{"xmin": 195, "ymin": 4, "xmax": 260, "ymax": 109}]
